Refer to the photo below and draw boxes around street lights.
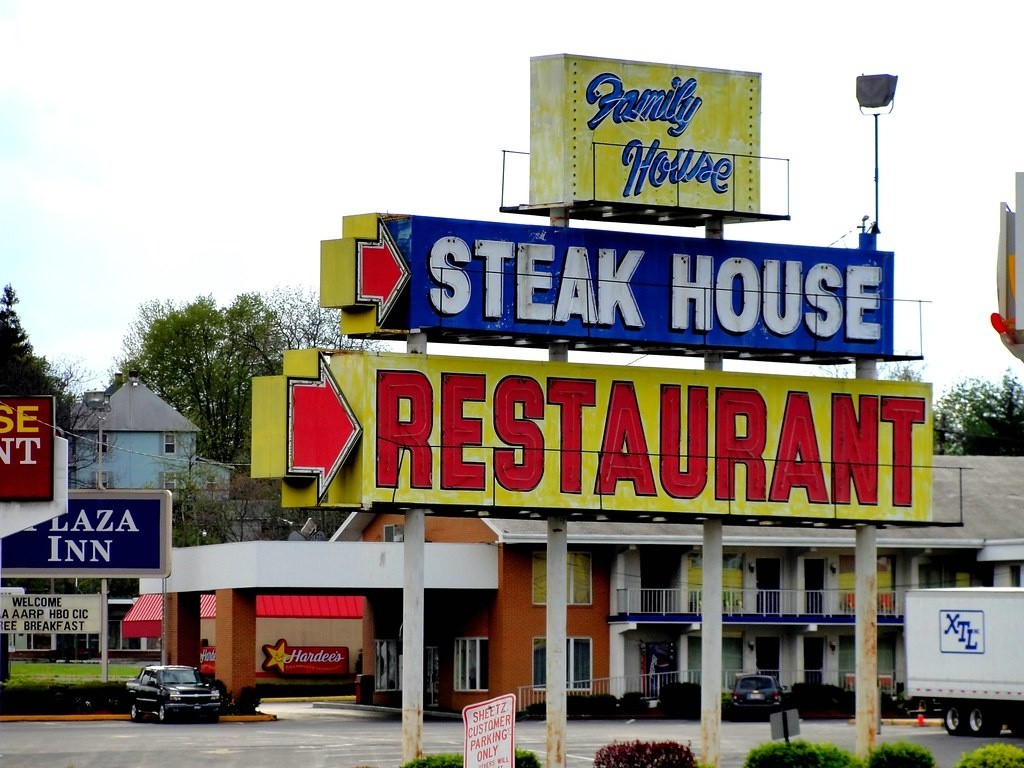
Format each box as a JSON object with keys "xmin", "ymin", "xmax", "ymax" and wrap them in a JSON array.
[
  {"xmin": 857, "ymin": 73, "xmax": 897, "ymax": 761},
  {"xmin": 82, "ymin": 392, "xmax": 112, "ymax": 682}
]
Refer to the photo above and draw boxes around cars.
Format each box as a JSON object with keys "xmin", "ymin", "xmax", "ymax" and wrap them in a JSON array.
[
  {"xmin": 731, "ymin": 675, "xmax": 788, "ymax": 716},
  {"xmin": 125, "ymin": 664, "xmax": 222, "ymax": 724}
]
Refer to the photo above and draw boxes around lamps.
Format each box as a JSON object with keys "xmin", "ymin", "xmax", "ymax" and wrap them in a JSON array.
[
  {"xmin": 749, "ymin": 562, "xmax": 756, "ymax": 573},
  {"xmin": 748, "ymin": 640, "xmax": 754, "ymax": 650},
  {"xmin": 830, "ymin": 563, "xmax": 837, "ymax": 574},
  {"xmin": 856, "ymin": 74, "xmax": 898, "ymax": 235},
  {"xmin": 829, "ymin": 640, "xmax": 836, "ymax": 651}
]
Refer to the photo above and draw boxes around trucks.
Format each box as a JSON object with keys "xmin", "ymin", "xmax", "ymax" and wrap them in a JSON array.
[{"xmin": 903, "ymin": 586, "xmax": 1024, "ymax": 738}]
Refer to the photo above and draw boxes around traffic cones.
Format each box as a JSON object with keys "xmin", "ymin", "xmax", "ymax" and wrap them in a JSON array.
[{"xmin": 915, "ymin": 701, "xmax": 929, "ymax": 727}]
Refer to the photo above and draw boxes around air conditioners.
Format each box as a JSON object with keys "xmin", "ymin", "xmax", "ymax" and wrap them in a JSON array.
[{"xmin": 383, "ymin": 524, "xmax": 404, "ymax": 541}]
